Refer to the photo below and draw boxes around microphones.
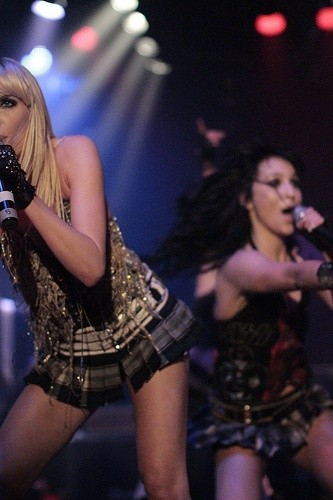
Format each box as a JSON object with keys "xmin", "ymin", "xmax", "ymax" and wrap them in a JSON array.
[
  {"xmin": 294, "ymin": 206, "xmax": 333, "ymax": 261},
  {"xmin": 0, "ymin": 178, "xmax": 19, "ymax": 230}
]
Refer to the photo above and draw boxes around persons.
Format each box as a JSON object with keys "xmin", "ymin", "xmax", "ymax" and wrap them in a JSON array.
[
  {"xmin": 149, "ymin": 141, "xmax": 333, "ymax": 499},
  {"xmin": 0, "ymin": 57, "xmax": 199, "ymax": 500}
]
